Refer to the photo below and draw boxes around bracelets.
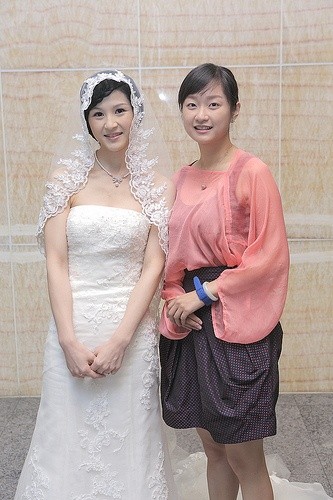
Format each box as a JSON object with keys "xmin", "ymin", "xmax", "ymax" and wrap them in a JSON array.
[
  {"xmin": 192, "ymin": 276, "xmax": 212, "ymax": 306},
  {"xmin": 202, "ymin": 281, "xmax": 218, "ymax": 301}
]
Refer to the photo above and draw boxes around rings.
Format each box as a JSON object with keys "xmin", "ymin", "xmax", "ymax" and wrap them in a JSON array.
[
  {"xmin": 104, "ymin": 372, "xmax": 111, "ymax": 376},
  {"xmin": 79, "ymin": 373, "xmax": 83, "ymax": 377}
]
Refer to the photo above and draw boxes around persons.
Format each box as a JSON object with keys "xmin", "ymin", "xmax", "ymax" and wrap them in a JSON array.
[
  {"xmin": 12, "ymin": 70, "xmax": 169, "ymax": 499},
  {"xmin": 159, "ymin": 62, "xmax": 289, "ymax": 500}
]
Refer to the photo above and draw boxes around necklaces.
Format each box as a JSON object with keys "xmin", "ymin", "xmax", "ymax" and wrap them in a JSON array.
[
  {"xmin": 199, "ymin": 145, "xmax": 233, "ymax": 190},
  {"xmin": 94, "ymin": 149, "xmax": 130, "ymax": 188}
]
